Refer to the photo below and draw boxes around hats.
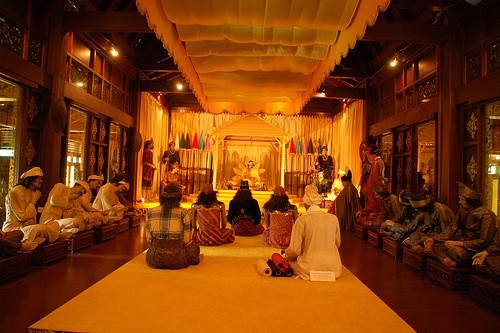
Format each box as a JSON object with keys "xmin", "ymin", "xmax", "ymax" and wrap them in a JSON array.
[
  {"xmin": 239, "ymin": 180, "xmax": 250, "ymax": 190},
  {"xmin": 201, "ymin": 184, "xmax": 214, "ymax": 195},
  {"xmin": 273, "ymin": 186, "xmax": 286, "ymax": 198},
  {"xmin": 303, "ymin": 185, "xmax": 322, "ymax": 206},
  {"xmin": 341, "ymin": 176, "xmax": 350, "ymax": 182}
]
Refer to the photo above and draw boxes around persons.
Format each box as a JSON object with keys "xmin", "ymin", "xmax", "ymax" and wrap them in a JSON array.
[
  {"xmin": 262, "ymin": 185, "xmax": 299, "ymax": 249},
  {"xmin": 2, "ymin": 165, "xmax": 73, "ymax": 245},
  {"xmin": 352, "ymin": 135, "xmax": 433, "ymax": 243},
  {"xmin": 145, "ymin": 180, "xmax": 204, "ymax": 270},
  {"xmin": 140, "ymin": 139, "xmax": 188, "ymax": 203},
  {"xmin": 191, "ymin": 183, "xmax": 236, "ymax": 246},
  {"xmin": 471, "ymin": 225, "xmax": 500, "ymax": 278},
  {"xmin": 73, "ymin": 174, "xmax": 116, "ymax": 229},
  {"xmin": 281, "ymin": 189, "xmax": 341, "ymax": 281},
  {"xmin": 92, "ymin": 175, "xmax": 136, "ymax": 222},
  {"xmin": 0, "ymin": 230, "xmax": 38, "ymax": 254},
  {"xmin": 240, "ymin": 160, "xmax": 261, "ymax": 185},
  {"xmin": 401, "ymin": 191, "xmax": 457, "ymax": 257},
  {"xmin": 314, "ymin": 145, "xmax": 335, "ymax": 197},
  {"xmin": 327, "ymin": 175, "xmax": 362, "ymax": 229},
  {"xmin": 227, "ymin": 180, "xmax": 265, "ymax": 237},
  {"xmin": 423, "ymin": 181, "xmax": 498, "ymax": 268},
  {"xmin": 38, "ymin": 179, "xmax": 94, "ymax": 234}
]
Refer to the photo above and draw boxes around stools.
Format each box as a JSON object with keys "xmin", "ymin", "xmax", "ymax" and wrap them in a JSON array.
[
  {"xmin": 352, "ymin": 222, "xmax": 368, "ymax": 239},
  {"xmin": 382, "ymin": 236, "xmax": 402, "ymax": 260},
  {"xmin": 69, "ymin": 230, "xmax": 95, "ymax": 252},
  {"xmin": 468, "ymin": 274, "xmax": 500, "ymax": 314},
  {"xmin": 367, "ymin": 229, "xmax": 383, "ymax": 250},
  {"xmin": 93, "ymin": 224, "xmax": 118, "ymax": 241},
  {"xmin": 402, "ymin": 245, "xmax": 426, "ymax": 273},
  {"xmin": 0, "ymin": 251, "xmax": 30, "ymax": 285},
  {"xmin": 125, "ymin": 215, "xmax": 141, "ymax": 227},
  {"xmin": 32, "ymin": 240, "xmax": 68, "ymax": 266},
  {"xmin": 114, "ymin": 218, "xmax": 130, "ymax": 233},
  {"xmin": 426, "ymin": 256, "xmax": 467, "ymax": 288}
]
